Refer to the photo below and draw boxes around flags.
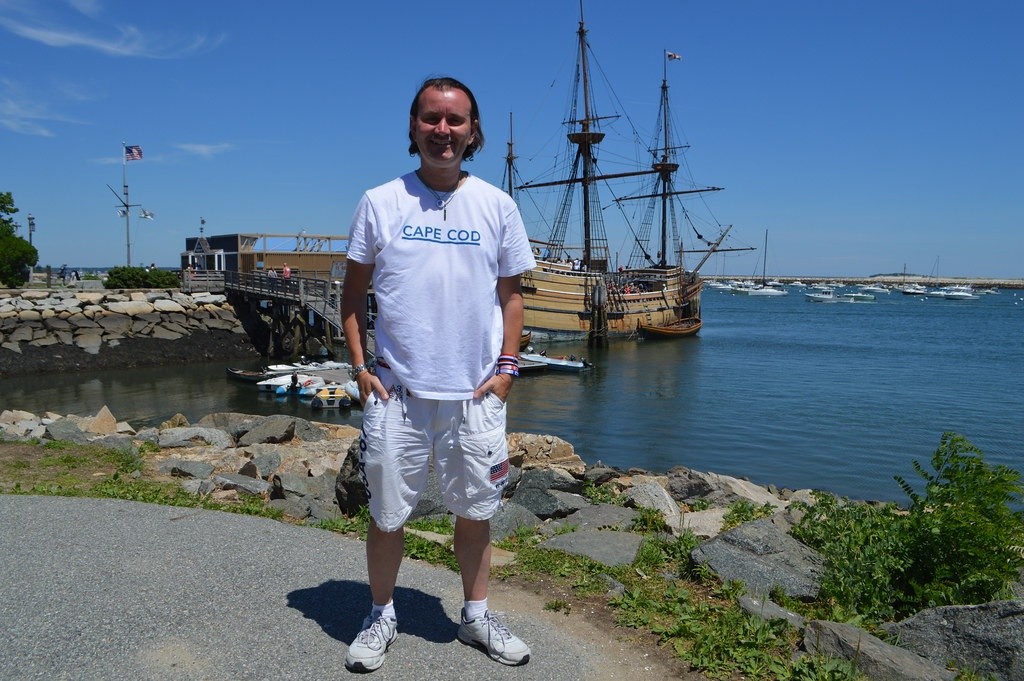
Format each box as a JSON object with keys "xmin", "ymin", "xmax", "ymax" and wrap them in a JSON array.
[
  {"xmin": 125, "ymin": 145, "xmax": 144, "ymax": 161},
  {"xmin": 668, "ymin": 53, "xmax": 681, "ymax": 61},
  {"xmin": 139, "ymin": 209, "xmax": 154, "ymax": 220}
]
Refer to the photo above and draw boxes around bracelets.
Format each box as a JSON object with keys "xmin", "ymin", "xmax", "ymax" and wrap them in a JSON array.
[{"xmin": 497, "ymin": 354, "xmax": 519, "ymax": 378}]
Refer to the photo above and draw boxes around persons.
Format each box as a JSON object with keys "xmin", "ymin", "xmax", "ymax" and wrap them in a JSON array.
[
  {"xmin": 532, "ymin": 246, "xmax": 587, "ymax": 276},
  {"xmin": 59, "ymin": 266, "xmax": 65, "ymax": 284},
  {"xmin": 658, "ymin": 259, "xmax": 667, "ymax": 269},
  {"xmin": 145, "ymin": 264, "xmax": 155, "ymax": 272},
  {"xmin": 300, "ymin": 355, "xmax": 310, "ymax": 365},
  {"xmin": 606, "ymin": 279, "xmax": 649, "ymax": 295},
  {"xmin": 289, "ymin": 373, "xmax": 298, "ymax": 388},
  {"xmin": 268, "ymin": 267, "xmax": 278, "ymax": 294},
  {"xmin": 70, "ymin": 270, "xmax": 80, "ymax": 281},
  {"xmin": 662, "ymin": 284, "xmax": 666, "ymax": 290},
  {"xmin": 186, "ymin": 263, "xmax": 203, "ymax": 278},
  {"xmin": 340, "ymin": 77, "xmax": 538, "ymax": 674},
  {"xmin": 282, "ymin": 263, "xmax": 291, "ymax": 292}
]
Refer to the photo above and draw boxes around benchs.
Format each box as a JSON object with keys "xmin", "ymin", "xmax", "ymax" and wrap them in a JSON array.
[
  {"xmin": 235, "ymin": 370, "xmax": 244, "ymax": 373},
  {"xmin": 75, "ymin": 280, "xmax": 104, "ymax": 290}
]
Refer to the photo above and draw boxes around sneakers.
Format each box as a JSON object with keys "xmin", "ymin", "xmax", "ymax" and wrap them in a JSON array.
[
  {"xmin": 345, "ymin": 611, "xmax": 397, "ymax": 671},
  {"xmin": 458, "ymin": 606, "xmax": 532, "ymax": 666}
]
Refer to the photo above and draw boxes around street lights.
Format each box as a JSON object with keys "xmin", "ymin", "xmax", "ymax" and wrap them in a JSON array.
[{"xmin": 29, "ymin": 212, "xmax": 35, "ymax": 285}]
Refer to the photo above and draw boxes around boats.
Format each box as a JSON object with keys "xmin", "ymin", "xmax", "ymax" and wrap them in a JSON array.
[
  {"xmin": 225, "ymin": 353, "xmax": 371, "ymax": 411},
  {"xmin": 805, "ymin": 290, "xmax": 844, "ymax": 303},
  {"xmin": 843, "ymin": 292, "xmax": 878, "ymax": 300},
  {"xmin": 700, "ymin": 229, "xmax": 789, "ymax": 296},
  {"xmin": 517, "ymin": 331, "xmax": 532, "ymax": 350},
  {"xmin": 942, "ymin": 284, "xmax": 981, "ymax": 299},
  {"xmin": 854, "ymin": 255, "xmax": 1003, "ymax": 297},
  {"xmin": 788, "ymin": 281, "xmax": 808, "ymax": 287},
  {"xmin": 807, "ymin": 281, "xmax": 846, "ymax": 292},
  {"xmin": 519, "ymin": 349, "xmax": 594, "ymax": 372},
  {"xmin": 516, "ymin": 357, "xmax": 549, "ymax": 369}
]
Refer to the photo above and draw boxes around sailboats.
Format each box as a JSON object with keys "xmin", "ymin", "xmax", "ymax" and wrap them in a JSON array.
[{"xmin": 219, "ymin": 0, "xmax": 759, "ymax": 361}]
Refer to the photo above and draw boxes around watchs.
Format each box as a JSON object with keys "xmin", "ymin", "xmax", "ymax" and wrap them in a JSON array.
[{"xmin": 348, "ymin": 365, "xmax": 368, "ymax": 382}]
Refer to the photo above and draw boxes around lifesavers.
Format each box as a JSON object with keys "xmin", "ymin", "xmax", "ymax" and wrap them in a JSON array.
[{"xmin": 304, "ymin": 380, "xmax": 314, "ymax": 386}]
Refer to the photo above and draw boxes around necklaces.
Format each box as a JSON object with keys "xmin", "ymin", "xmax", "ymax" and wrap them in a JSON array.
[{"xmin": 417, "ymin": 168, "xmax": 462, "ymax": 220}]
